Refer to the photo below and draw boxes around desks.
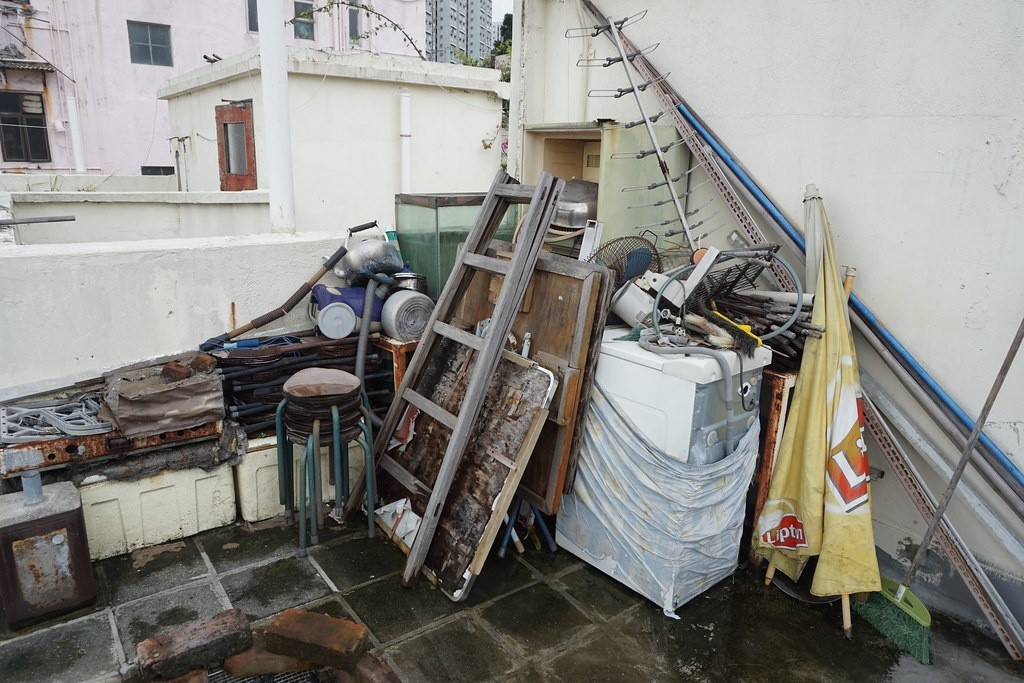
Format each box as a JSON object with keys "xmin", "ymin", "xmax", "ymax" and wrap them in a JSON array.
[{"xmin": 351, "ymin": 236, "xmax": 616, "ymax": 603}]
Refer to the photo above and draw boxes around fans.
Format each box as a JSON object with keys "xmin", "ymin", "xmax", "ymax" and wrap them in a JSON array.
[{"xmin": 582, "ymin": 236, "xmax": 664, "ymax": 332}]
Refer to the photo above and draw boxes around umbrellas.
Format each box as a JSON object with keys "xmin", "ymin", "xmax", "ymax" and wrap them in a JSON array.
[{"xmin": 760, "ymin": 182, "xmax": 882, "ymax": 630}]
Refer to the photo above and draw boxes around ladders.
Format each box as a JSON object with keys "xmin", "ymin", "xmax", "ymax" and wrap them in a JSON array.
[{"xmin": 342, "ymin": 170, "xmax": 566, "ymax": 587}]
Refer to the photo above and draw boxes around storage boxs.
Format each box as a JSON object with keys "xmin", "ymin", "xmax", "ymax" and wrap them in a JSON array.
[
  {"xmin": 395, "ymin": 191, "xmax": 519, "ymax": 305},
  {"xmin": 76, "ymin": 446, "xmax": 237, "ymax": 563},
  {"xmin": 236, "ymin": 416, "xmax": 369, "ymax": 522}
]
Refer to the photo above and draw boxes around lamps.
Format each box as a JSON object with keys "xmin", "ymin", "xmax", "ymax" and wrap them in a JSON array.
[{"xmin": 53, "ymin": 120, "xmax": 65, "ymax": 134}]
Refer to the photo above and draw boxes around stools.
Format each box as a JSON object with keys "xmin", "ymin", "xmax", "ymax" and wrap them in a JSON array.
[{"xmin": 275, "ymin": 367, "xmax": 379, "ymax": 559}]
